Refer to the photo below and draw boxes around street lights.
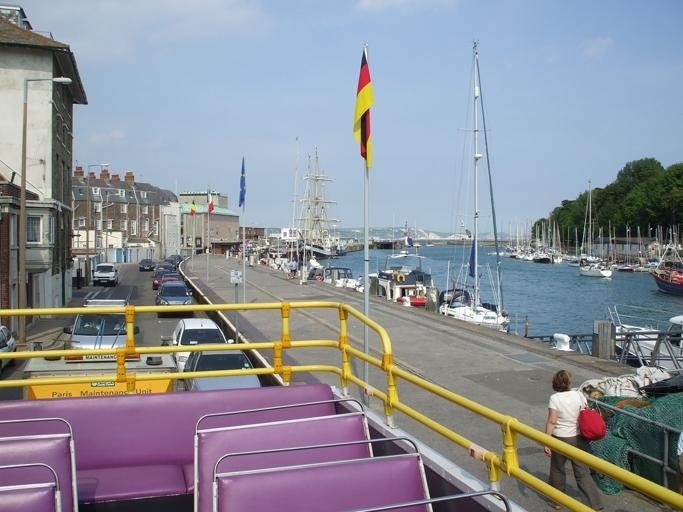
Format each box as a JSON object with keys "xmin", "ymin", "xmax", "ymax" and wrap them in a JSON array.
[
  {"xmin": 14, "ymin": 75, "xmax": 73, "ymax": 354},
  {"xmin": 85, "ymin": 163, "xmax": 109, "ymax": 285}
]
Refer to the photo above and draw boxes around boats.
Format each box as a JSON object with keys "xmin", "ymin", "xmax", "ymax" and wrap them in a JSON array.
[
  {"xmin": 253, "ymin": 229, "xmax": 439, "ymax": 310},
  {"xmin": 605, "ymin": 305, "xmax": 683, "ymax": 376}
]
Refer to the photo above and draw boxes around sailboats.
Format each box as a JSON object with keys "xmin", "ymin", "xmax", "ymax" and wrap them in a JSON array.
[
  {"xmin": 487, "ymin": 179, "xmax": 683, "ymax": 297},
  {"xmin": 287, "ymin": 138, "xmax": 346, "ymax": 258},
  {"xmin": 440, "ymin": 39, "xmax": 510, "ymax": 336}
]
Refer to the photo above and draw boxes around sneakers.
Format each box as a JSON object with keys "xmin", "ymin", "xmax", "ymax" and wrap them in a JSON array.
[{"xmin": 548, "ymin": 500, "xmax": 561, "ymax": 510}]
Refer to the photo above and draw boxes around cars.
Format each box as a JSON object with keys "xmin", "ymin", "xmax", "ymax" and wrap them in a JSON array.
[
  {"xmin": 0, "ymin": 326, "xmax": 18, "ymax": 374},
  {"xmin": 63, "ymin": 244, "xmax": 257, "ymax": 392}
]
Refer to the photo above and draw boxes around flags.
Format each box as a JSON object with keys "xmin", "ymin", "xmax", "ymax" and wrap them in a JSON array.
[
  {"xmin": 190, "ymin": 200, "xmax": 196, "ymax": 216},
  {"xmin": 353, "ymin": 49, "xmax": 375, "ymax": 170},
  {"xmin": 238, "ymin": 157, "xmax": 246, "ymax": 207},
  {"xmin": 206, "ymin": 188, "xmax": 214, "ymax": 215}
]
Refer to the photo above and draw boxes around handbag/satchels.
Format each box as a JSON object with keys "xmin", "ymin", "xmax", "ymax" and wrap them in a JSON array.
[{"xmin": 578, "ymin": 407, "xmax": 607, "ymax": 441}]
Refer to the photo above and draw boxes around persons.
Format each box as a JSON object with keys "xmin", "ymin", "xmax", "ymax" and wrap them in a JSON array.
[
  {"xmin": 543, "ymin": 369, "xmax": 606, "ymax": 510},
  {"xmin": 676, "ymin": 431, "xmax": 683, "ymax": 494}
]
